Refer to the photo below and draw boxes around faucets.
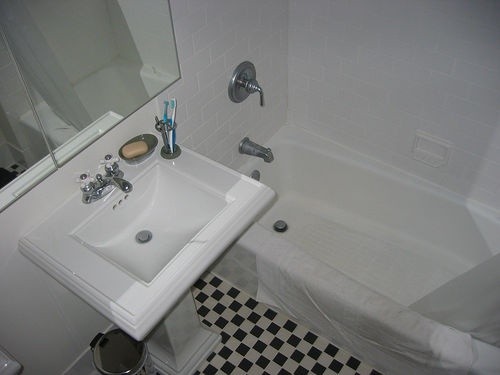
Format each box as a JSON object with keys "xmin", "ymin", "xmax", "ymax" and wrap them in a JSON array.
[
  {"xmin": 238, "ymin": 137, "xmax": 274, "ymax": 163},
  {"xmin": 79, "ymin": 154, "xmax": 133, "ymax": 204}
]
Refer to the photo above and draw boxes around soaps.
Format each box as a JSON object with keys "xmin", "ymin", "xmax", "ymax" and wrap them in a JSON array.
[{"xmin": 121, "ymin": 141, "xmax": 148, "ymax": 159}]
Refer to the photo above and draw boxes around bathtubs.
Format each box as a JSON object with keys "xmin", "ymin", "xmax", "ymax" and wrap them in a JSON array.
[{"xmin": 209, "ymin": 125, "xmax": 500, "ymax": 375}]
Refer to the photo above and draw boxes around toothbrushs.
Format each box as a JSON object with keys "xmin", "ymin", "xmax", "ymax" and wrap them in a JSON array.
[
  {"xmin": 169, "ymin": 97, "xmax": 177, "ymax": 153},
  {"xmin": 163, "ymin": 99, "xmax": 169, "ymax": 142}
]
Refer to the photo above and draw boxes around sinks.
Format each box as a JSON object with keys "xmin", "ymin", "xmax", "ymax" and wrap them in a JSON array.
[{"xmin": 18, "ymin": 135, "xmax": 279, "ymax": 342}]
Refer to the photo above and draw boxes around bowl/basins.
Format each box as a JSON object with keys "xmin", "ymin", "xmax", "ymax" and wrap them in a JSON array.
[{"xmin": 118, "ymin": 133, "xmax": 159, "ymax": 165}]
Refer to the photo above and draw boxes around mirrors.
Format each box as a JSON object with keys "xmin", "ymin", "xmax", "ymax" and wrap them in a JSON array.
[
  {"xmin": 0, "ymin": 0, "xmax": 181, "ymax": 168},
  {"xmin": 0, "ymin": 35, "xmax": 57, "ymax": 213}
]
